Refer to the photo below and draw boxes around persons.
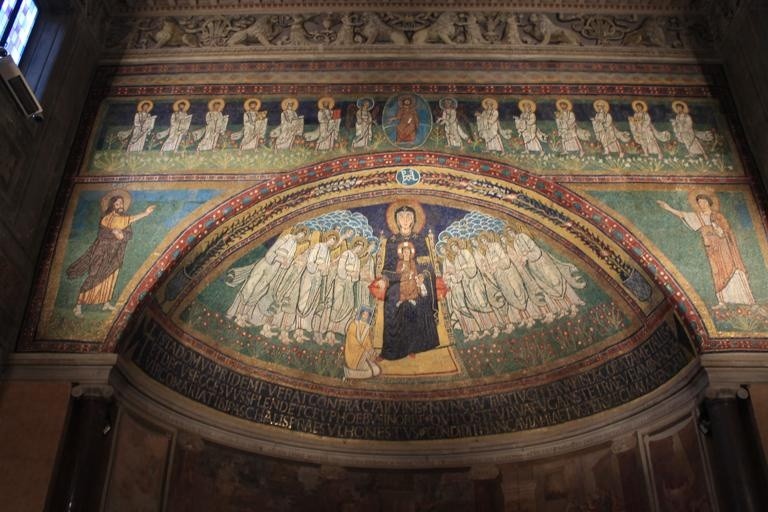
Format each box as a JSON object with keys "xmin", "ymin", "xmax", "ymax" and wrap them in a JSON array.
[
  {"xmin": 65, "ymin": 189, "xmax": 155, "ymax": 317},
  {"xmin": 655, "ymin": 188, "xmax": 757, "ymax": 309}
]
